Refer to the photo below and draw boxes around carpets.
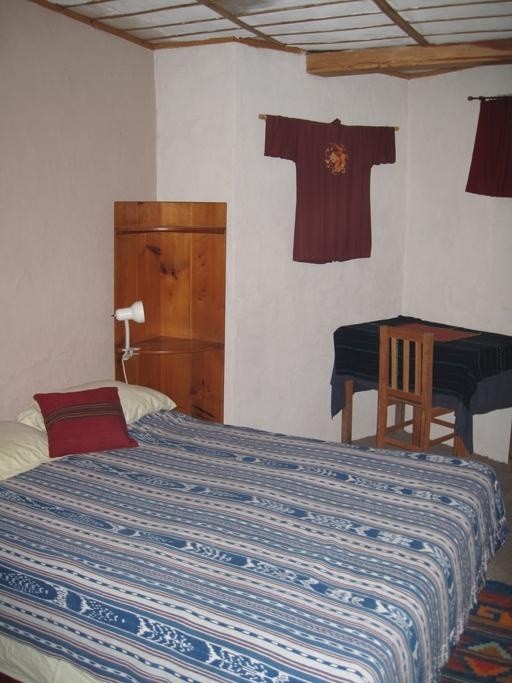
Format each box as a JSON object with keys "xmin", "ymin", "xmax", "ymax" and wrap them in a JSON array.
[{"xmin": 438, "ymin": 578, "xmax": 511, "ymax": 683}]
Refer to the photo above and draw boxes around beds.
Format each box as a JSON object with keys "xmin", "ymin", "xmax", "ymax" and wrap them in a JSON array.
[{"xmin": 0, "ymin": 409, "xmax": 509, "ymax": 682}]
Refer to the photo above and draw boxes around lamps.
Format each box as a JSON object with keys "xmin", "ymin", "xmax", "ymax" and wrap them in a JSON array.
[{"xmin": 114, "ymin": 300, "xmax": 147, "ymax": 385}]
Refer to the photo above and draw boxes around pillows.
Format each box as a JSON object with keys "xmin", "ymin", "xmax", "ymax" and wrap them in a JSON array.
[
  {"xmin": 18, "ymin": 378, "xmax": 176, "ymax": 432},
  {"xmin": 33, "ymin": 387, "xmax": 138, "ymax": 457},
  {"xmin": 0, "ymin": 420, "xmax": 66, "ymax": 481}
]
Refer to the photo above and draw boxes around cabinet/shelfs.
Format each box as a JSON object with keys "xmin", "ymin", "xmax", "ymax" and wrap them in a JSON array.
[{"xmin": 113, "ymin": 200, "xmax": 227, "ymax": 423}]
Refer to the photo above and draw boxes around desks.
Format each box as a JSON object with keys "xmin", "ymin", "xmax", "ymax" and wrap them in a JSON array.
[{"xmin": 331, "ymin": 315, "xmax": 511, "ymax": 465}]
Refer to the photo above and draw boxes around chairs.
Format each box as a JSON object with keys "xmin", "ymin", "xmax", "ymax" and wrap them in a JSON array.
[{"xmin": 377, "ymin": 324, "xmax": 457, "ymax": 456}]
